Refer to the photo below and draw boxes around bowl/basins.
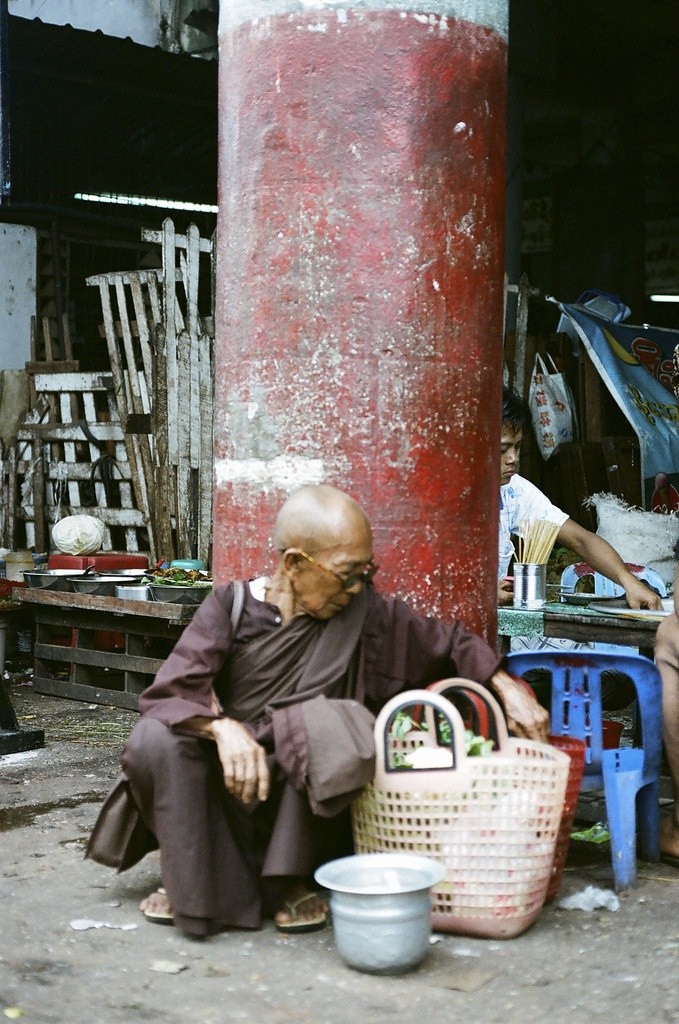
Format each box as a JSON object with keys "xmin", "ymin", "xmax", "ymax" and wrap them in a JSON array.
[{"xmin": 20, "ymin": 569, "xmax": 213, "ymax": 605}]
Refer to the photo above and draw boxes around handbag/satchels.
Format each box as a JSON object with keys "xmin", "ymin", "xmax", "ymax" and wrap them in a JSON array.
[{"xmin": 528, "ymin": 352, "xmax": 580, "ymax": 460}]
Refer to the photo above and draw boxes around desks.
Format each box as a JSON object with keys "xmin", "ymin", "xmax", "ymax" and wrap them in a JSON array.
[{"xmin": 496, "ymin": 604, "xmax": 666, "ymax": 747}]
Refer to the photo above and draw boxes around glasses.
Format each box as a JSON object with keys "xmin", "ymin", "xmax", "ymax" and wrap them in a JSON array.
[{"xmin": 279, "ymin": 548, "xmax": 379, "ymax": 590}]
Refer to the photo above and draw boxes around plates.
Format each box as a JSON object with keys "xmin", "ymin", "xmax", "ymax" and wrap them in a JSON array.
[
  {"xmin": 543, "ymin": 585, "xmax": 572, "ymax": 594},
  {"xmin": 555, "ymin": 592, "xmax": 611, "ymax": 602}
]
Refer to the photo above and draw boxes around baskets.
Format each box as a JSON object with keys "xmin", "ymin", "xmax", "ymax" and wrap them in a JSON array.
[{"xmin": 349, "ymin": 677, "xmax": 586, "ymax": 938}]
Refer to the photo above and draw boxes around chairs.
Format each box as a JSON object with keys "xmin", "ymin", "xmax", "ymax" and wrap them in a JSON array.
[
  {"xmin": 500, "ymin": 652, "xmax": 664, "ymax": 892},
  {"xmin": 561, "ymin": 562, "xmax": 667, "ymax": 602}
]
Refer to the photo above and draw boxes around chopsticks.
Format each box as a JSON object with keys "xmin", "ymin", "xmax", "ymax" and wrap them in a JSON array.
[{"xmin": 507, "ymin": 518, "xmax": 561, "ymax": 563}]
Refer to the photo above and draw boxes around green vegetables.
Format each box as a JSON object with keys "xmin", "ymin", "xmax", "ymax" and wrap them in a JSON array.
[{"xmin": 140, "ymin": 568, "xmax": 202, "ymax": 587}]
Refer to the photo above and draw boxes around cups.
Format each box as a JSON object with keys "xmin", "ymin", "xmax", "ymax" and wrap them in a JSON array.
[{"xmin": 513, "ymin": 566, "xmax": 543, "ymax": 611}]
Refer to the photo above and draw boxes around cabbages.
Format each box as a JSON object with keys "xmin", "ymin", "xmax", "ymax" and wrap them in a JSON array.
[{"xmin": 52, "ymin": 514, "xmax": 106, "ymax": 555}]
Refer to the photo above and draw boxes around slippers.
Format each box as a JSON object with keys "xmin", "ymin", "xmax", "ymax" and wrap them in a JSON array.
[
  {"xmin": 144, "ymin": 887, "xmax": 176, "ymax": 925},
  {"xmin": 276, "ymin": 891, "xmax": 326, "ymax": 933},
  {"xmin": 660, "ymin": 853, "xmax": 679, "ymax": 868}
]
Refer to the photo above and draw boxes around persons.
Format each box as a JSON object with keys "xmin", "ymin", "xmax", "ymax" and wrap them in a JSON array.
[
  {"xmin": 121, "ymin": 484, "xmax": 552, "ymax": 936},
  {"xmin": 497, "ymin": 385, "xmax": 665, "ymax": 711},
  {"xmin": 654, "ymin": 538, "xmax": 679, "ymax": 868}
]
